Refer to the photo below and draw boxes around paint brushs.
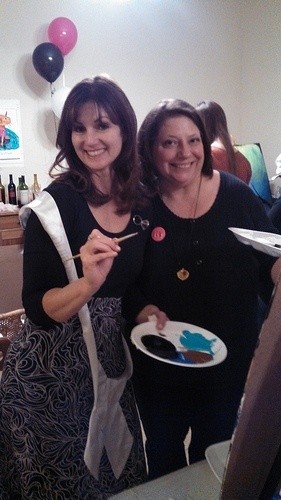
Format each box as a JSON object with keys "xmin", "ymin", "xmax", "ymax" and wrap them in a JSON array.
[{"xmin": 63, "ymin": 232, "xmax": 138, "ymax": 262}]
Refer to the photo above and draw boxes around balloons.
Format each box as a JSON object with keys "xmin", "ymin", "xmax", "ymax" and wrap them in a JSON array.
[{"xmin": 33, "ymin": 18, "xmax": 77, "ymax": 84}]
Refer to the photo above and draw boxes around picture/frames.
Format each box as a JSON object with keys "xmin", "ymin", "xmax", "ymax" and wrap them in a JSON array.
[{"xmin": 0, "ymin": 99, "xmax": 23, "ymax": 159}]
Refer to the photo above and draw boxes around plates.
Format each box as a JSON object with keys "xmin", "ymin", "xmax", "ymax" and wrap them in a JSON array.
[
  {"xmin": 130, "ymin": 321, "xmax": 227, "ymax": 368},
  {"xmin": 205, "ymin": 440, "xmax": 230, "ymax": 483},
  {"xmin": 228, "ymin": 227, "xmax": 281, "ymax": 257}
]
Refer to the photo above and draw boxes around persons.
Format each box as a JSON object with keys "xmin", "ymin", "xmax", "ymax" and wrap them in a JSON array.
[
  {"xmin": 195, "ymin": 100, "xmax": 252, "ymax": 184},
  {"xmin": 0, "ymin": 75, "xmax": 171, "ymax": 500},
  {"xmin": 137, "ymin": 98, "xmax": 281, "ymax": 480}
]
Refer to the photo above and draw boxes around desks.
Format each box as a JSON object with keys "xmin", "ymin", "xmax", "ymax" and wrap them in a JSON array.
[{"xmin": 0, "ymin": 203, "xmax": 29, "ymax": 318}]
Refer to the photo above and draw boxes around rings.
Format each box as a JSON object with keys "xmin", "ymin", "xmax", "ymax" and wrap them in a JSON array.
[{"xmin": 88, "ymin": 235, "xmax": 93, "ymax": 241}]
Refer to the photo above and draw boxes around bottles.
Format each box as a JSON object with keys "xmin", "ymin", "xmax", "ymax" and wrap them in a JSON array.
[
  {"xmin": 8, "ymin": 174, "xmax": 16, "ymax": 205},
  {"xmin": 32, "ymin": 174, "xmax": 40, "ymax": 201},
  {"xmin": 0, "ymin": 175, "xmax": 5, "ymax": 204},
  {"xmin": 17, "ymin": 176, "xmax": 29, "ymax": 207}
]
